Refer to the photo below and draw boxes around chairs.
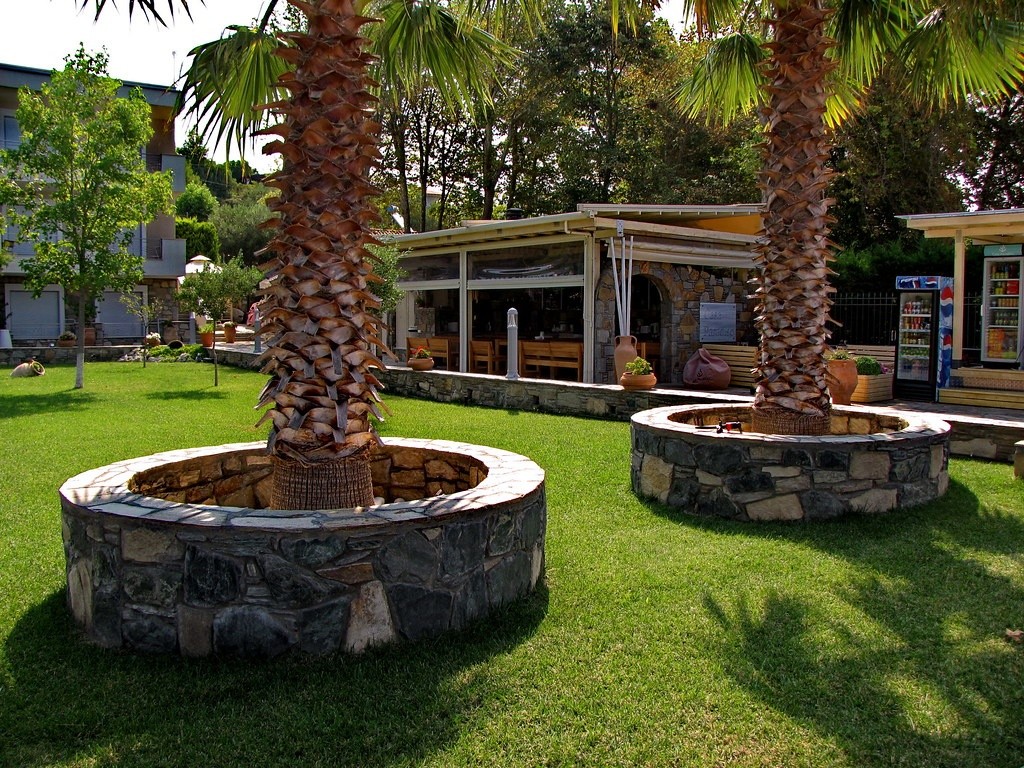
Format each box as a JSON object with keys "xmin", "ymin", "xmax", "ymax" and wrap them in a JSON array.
[{"xmin": 471, "ymin": 338, "xmax": 507, "ymax": 375}]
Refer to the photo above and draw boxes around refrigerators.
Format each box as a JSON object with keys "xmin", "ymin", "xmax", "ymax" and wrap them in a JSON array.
[
  {"xmin": 892, "ymin": 276, "xmax": 954, "ymax": 402},
  {"xmin": 980, "ymin": 244, "xmax": 1024, "ymax": 368}
]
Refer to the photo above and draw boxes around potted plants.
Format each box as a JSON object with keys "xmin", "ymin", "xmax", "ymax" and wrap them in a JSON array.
[
  {"xmin": 63, "ymin": 299, "xmax": 101, "ymax": 346},
  {"xmin": 826, "ymin": 351, "xmax": 894, "ymax": 405},
  {"xmin": 619, "ymin": 358, "xmax": 657, "ymax": 390}
]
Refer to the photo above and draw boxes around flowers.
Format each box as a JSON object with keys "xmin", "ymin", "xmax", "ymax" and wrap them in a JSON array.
[
  {"xmin": 223, "ymin": 321, "xmax": 238, "ymax": 328},
  {"xmin": 58, "ymin": 331, "xmax": 77, "ymax": 340},
  {"xmin": 145, "ymin": 332, "xmax": 161, "ymax": 339},
  {"xmin": 411, "ymin": 345, "xmax": 431, "ymax": 359}
]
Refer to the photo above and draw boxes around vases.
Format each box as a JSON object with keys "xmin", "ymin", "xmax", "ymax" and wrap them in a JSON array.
[
  {"xmin": 408, "ymin": 358, "xmax": 435, "ymax": 370},
  {"xmin": 225, "ymin": 327, "xmax": 236, "ymax": 343},
  {"xmin": 142, "ymin": 338, "xmax": 161, "ymax": 346},
  {"xmin": 196, "ymin": 321, "xmax": 217, "ymax": 347},
  {"xmin": 57, "ymin": 340, "xmax": 75, "ymax": 346}
]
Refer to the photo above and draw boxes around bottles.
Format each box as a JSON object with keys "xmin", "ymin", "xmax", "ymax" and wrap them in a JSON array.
[
  {"xmin": 902, "ymin": 299, "xmax": 931, "ymax": 371},
  {"xmin": 989, "ymin": 263, "xmax": 1019, "ymax": 325}
]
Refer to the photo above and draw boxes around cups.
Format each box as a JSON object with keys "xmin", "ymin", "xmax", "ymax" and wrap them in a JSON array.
[{"xmin": 540, "ymin": 332, "xmax": 544, "ymax": 339}]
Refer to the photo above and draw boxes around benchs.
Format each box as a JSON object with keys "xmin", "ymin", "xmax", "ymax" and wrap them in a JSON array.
[
  {"xmin": 702, "ymin": 344, "xmax": 763, "ymax": 394},
  {"xmin": 406, "ymin": 335, "xmax": 459, "ymax": 372},
  {"xmin": 518, "ymin": 338, "xmax": 660, "ymax": 383},
  {"xmin": 846, "ymin": 344, "xmax": 895, "ymax": 372}
]
